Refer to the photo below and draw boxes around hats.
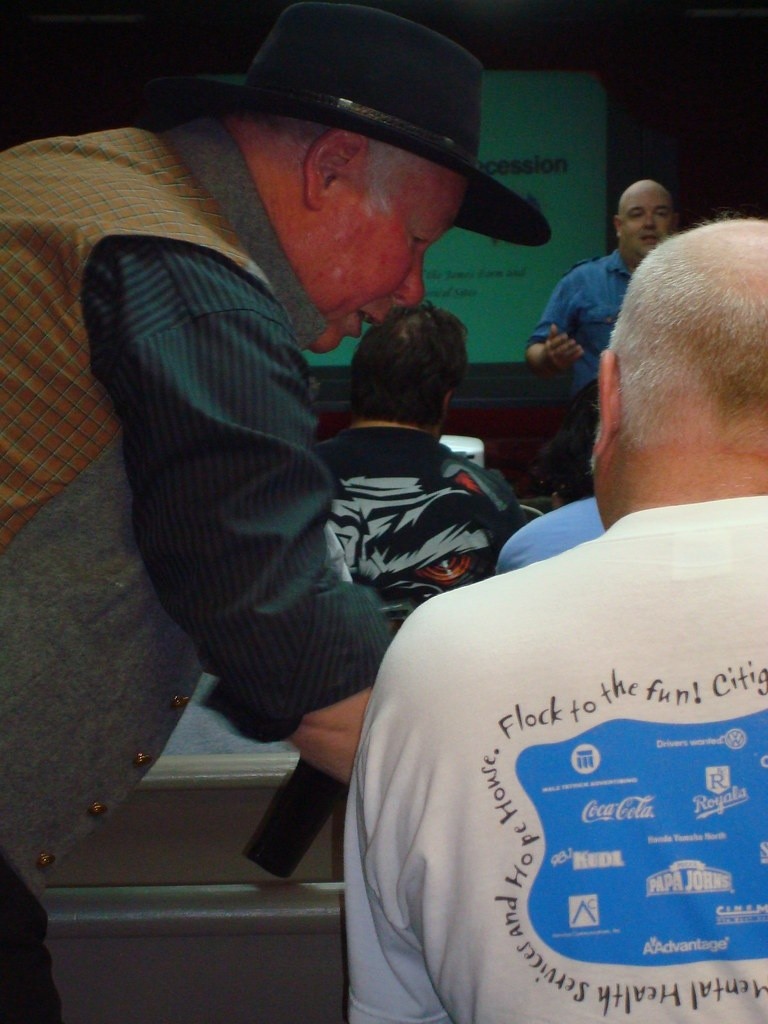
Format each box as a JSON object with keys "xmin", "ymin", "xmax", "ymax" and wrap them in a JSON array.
[{"xmin": 144, "ymin": 1, "xmax": 552, "ymax": 254}]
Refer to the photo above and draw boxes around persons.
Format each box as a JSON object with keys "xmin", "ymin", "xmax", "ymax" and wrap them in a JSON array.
[
  {"xmin": 1, "ymin": 0, "xmax": 554, "ymax": 1024},
  {"xmin": 492, "ymin": 494, "xmax": 606, "ymax": 581},
  {"xmin": 525, "ymin": 176, "xmax": 677, "ymax": 501},
  {"xmin": 311, "ymin": 303, "xmax": 527, "ymax": 646},
  {"xmin": 348, "ymin": 218, "xmax": 768, "ymax": 1024}
]
